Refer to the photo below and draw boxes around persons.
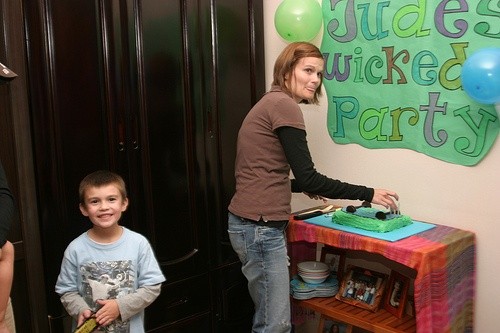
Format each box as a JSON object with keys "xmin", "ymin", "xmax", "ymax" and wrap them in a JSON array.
[
  {"xmin": 55, "ymin": 171, "xmax": 166, "ymax": 333},
  {"xmin": 343, "ymin": 278, "xmax": 377, "ymax": 305},
  {"xmin": 227, "ymin": 42, "xmax": 400, "ymax": 333},
  {"xmin": 0, "ymin": 160, "xmax": 16, "ymax": 333}
]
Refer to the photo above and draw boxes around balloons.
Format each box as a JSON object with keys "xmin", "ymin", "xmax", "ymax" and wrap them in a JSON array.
[
  {"xmin": 461, "ymin": 48, "xmax": 500, "ymax": 104},
  {"xmin": 275, "ymin": 0, "xmax": 323, "ymax": 42}
]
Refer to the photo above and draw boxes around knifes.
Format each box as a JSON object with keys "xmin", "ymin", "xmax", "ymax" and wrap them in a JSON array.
[{"xmin": 293, "ymin": 208, "xmax": 337, "ymax": 221}]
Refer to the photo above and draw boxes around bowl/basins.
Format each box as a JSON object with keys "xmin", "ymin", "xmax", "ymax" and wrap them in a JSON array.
[{"xmin": 296, "ymin": 261, "xmax": 330, "ymax": 284}]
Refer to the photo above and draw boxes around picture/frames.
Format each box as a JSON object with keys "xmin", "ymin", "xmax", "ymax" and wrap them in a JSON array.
[
  {"xmin": 383, "ymin": 269, "xmax": 410, "ymax": 319},
  {"xmin": 317, "ymin": 314, "xmax": 353, "ymax": 333},
  {"xmin": 320, "ymin": 247, "xmax": 345, "ymax": 285},
  {"xmin": 335, "ymin": 264, "xmax": 389, "ymax": 313}
]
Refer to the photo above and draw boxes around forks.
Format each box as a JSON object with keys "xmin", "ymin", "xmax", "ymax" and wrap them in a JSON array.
[{"xmin": 299, "ymin": 204, "xmax": 334, "ymax": 214}]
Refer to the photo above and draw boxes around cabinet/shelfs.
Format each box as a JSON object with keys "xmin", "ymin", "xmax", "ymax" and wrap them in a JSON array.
[
  {"xmin": 287, "ymin": 205, "xmax": 476, "ymax": 333},
  {"xmin": 0, "ymin": 0, "xmax": 265, "ymax": 333}
]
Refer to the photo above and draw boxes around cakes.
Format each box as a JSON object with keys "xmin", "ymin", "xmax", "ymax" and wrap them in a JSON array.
[{"xmin": 332, "ymin": 201, "xmax": 413, "ymax": 233}]
